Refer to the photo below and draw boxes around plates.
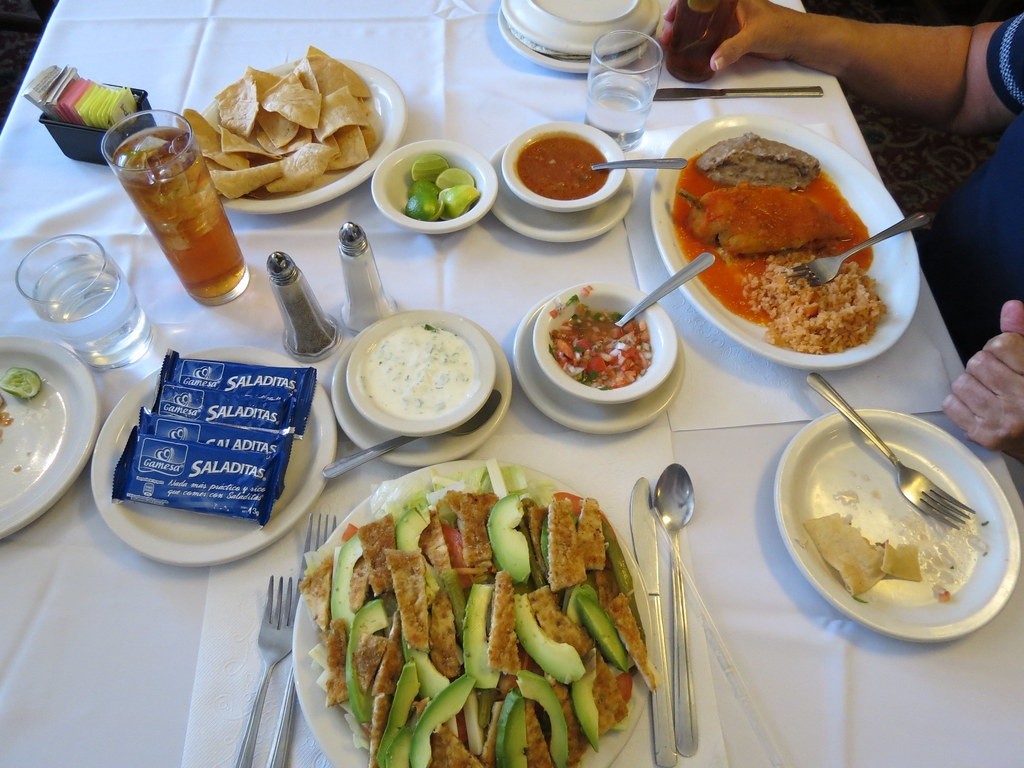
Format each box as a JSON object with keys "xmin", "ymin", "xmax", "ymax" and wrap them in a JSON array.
[
  {"xmin": 0, "ymin": 335, "xmax": 98, "ymax": 541},
  {"xmin": 331, "ymin": 309, "xmax": 515, "ymax": 468},
  {"xmin": 644, "ymin": 112, "xmax": 920, "ymax": 371},
  {"xmin": 290, "ymin": 457, "xmax": 658, "ymax": 767},
  {"xmin": 512, "ymin": 285, "xmax": 684, "ymax": 436},
  {"xmin": 496, "ymin": 0, "xmax": 663, "ymax": 71},
  {"xmin": 774, "ymin": 407, "xmax": 1022, "ymax": 643},
  {"xmin": 89, "ymin": 339, "xmax": 339, "ymax": 570},
  {"xmin": 195, "ymin": 56, "xmax": 404, "ymax": 211},
  {"xmin": 490, "ymin": 139, "xmax": 634, "ymax": 243}
]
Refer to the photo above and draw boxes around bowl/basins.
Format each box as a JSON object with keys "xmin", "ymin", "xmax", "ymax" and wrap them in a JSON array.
[
  {"xmin": 530, "ymin": 279, "xmax": 677, "ymax": 404},
  {"xmin": 371, "ymin": 139, "xmax": 502, "ymax": 234},
  {"xmin": 500, "ymin": 121, "xmax": 626, "ymax": 210},
  {"xmin": 344, "ymin": 309, "xmax": 494, "ymax": 437}
]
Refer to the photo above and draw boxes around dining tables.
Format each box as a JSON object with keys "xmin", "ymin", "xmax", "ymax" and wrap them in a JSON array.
[{"xmin": 0, "ymin": 0, "xmax": 1024, "ymax": 768}]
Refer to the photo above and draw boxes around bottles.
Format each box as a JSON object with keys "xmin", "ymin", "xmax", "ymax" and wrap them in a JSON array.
[
  {"xmin": 265, "ymin": 227, "xmax": 403, "ymax": 362},
  {"xmin": 665, "ymin": 0, "xmax": 734, "ymax": 83}
]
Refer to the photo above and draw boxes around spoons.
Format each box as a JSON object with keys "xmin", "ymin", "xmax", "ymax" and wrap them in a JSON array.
[
  {"xmin": 650, "ymin": 464, "xmax": 697, "ymax": 755},
  {"xmin": 321, "ymin": 390, "xmax": 502, "ymax": 480}
]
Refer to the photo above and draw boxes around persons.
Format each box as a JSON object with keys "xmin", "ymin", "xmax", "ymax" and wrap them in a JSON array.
[{"xmin": 660, "ymin": 0, "xmax": 1024, "ymax": 462}]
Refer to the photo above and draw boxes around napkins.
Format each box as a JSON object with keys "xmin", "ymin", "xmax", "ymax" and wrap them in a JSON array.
[
  {"xmin": 181, "ymin": 408, "xmax": 673, "ymax": 768},
  {"xmin": 618, "ymin": 121, "xmax": 953, "ymax": 433}
]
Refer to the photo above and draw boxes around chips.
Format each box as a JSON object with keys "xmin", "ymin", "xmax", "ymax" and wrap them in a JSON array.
[{"xmin": 180, "ymin": 45, "xmax": 377, "ymax": 198}]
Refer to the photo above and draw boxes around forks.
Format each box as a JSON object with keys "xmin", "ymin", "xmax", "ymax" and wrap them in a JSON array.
[
  {"xmin": 228, "ymin": 500, "xmax": 344, "ymax": 768},
  {"xmin": 780, "ymin": 212, "xmax": 931, "ymax": 288},
  {"xmin": 803, "ymin": 369, "xmax": 977, "ymax": 532}
]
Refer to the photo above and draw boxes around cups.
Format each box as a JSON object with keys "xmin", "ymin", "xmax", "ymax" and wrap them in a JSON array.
[
  {"xmin": 100, "ymin": 108, "xmax": 251, "ymax": 306},
  {"xmin": 584, "ymin": 31, "xmax": 665, "ymax": 150},
  {"xmin": 15, "ymin": 233, "xmax": 153, "ymax": 368}
]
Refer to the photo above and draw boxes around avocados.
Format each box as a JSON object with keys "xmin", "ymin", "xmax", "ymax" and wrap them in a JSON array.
[{"xmin": 328, "ymin": 494, "xmax": 631, "ymax": 768}]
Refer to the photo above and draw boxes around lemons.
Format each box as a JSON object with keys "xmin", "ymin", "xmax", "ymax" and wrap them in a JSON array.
[
  {"xmin": 405, "ymin": 155, "xmax": 480, "ymax": 221},
  {"xmin": 0, "ymin": 367, "xmax": 42, "ymax": 400}
]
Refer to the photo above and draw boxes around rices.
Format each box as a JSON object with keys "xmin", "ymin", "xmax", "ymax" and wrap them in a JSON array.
[{"xmin": 743, "ymin": 252, "xmax": 884, "ymax": 355}]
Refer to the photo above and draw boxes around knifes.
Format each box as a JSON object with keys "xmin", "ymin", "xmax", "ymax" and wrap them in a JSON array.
[
  {"xmin": 652, "ymin": 86, "xmax": 826, "ymax": 104},
  {"xmin": 625, "ymin": 477, "xmax": 681, "ymax": 768}
]
